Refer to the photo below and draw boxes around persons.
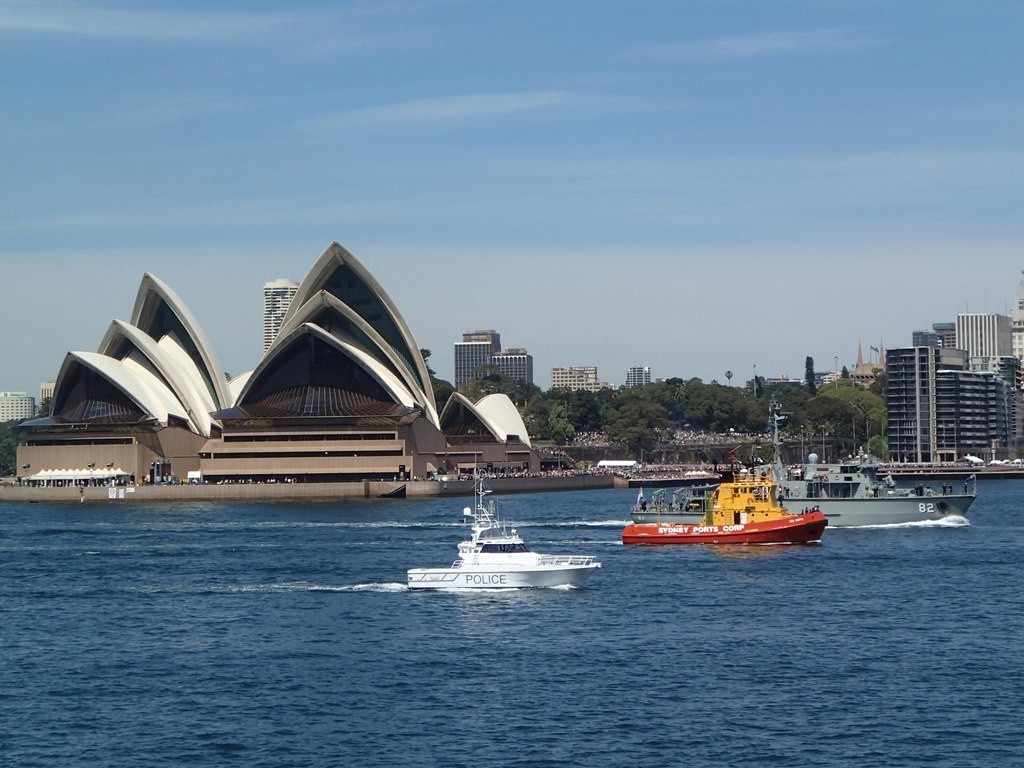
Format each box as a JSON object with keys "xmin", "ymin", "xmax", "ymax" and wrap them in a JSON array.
[
  {"xmin": 12, "ymin": 473, "xmax": 301, "ymax": 488},
  {"xmin": 394, "ymin": 430, "xmax": 968, "ymax": 515}
]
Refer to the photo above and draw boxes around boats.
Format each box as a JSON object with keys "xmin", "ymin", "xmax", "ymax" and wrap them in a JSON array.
[
  {"xmin": 405, "ymin": 451, "xmax": 604, "ymax": 591},
  {"xmin": 627, "ymin": 392, "xmax": 980, "ymax": 532},
  {"xmin": 622, "ymin": 449, "xmax": 829, "ymax": 547}
]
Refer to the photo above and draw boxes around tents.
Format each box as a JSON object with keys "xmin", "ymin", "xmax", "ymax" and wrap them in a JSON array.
[{"xmin": 21, "ymin": 467, "xmax": 131, "ymax": 487}]
